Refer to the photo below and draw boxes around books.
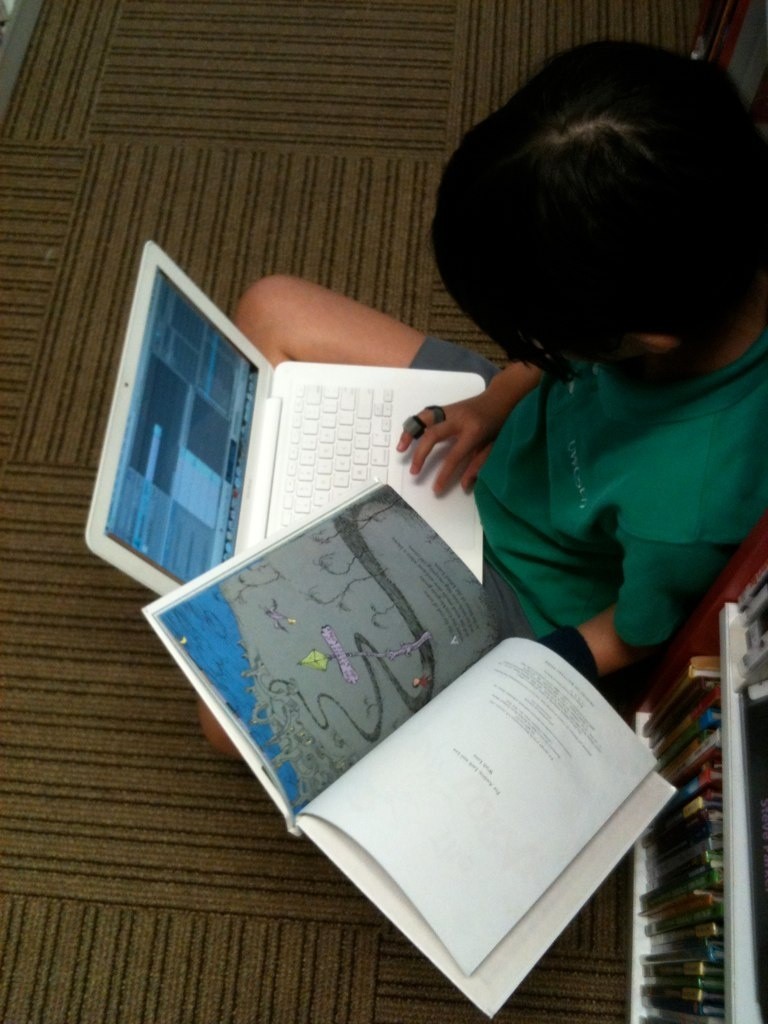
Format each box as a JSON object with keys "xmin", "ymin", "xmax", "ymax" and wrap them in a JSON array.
[{"xmin": 143, "ymin": 475, "xmax": 768, "ymax": 1023}]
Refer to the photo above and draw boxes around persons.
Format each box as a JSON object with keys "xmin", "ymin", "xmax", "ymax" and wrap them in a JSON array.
[{"xmin": 198, "ymin": 40, "xmax": 768, "ymax": 767}]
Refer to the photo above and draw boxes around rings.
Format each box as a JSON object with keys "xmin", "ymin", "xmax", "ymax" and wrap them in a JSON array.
[
  {"xmin": 425, "ymin": 405, "xmax": 447, "ymax": 424},
  {"xmin": 403, "ymin": 416, "xmax": 427, "ymax": 438}
]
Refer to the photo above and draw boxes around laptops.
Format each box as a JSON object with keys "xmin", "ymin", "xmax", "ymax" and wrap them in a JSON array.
[{"xmin": 81, "ymin": 236, "xmax": 487, "ymax": 599}]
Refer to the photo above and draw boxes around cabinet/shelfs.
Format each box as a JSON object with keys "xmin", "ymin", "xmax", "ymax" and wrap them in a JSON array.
[{"xmin": 627, "ymin": 510, "xmax": 768, "ymax": 1024}]
